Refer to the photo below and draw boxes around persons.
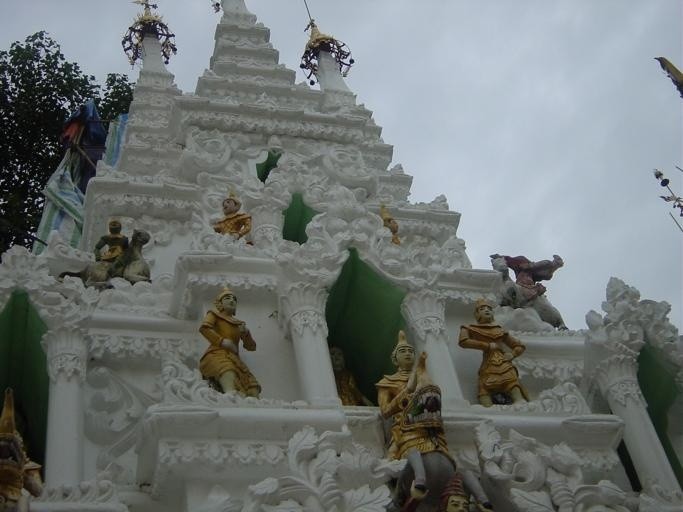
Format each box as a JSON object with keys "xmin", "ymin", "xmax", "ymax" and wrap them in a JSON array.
[
  {"xmin": 327, "ymin": 338, "xmax": 377, "ymax": 406},
  {"xmin": 456, "ymin": 297, "xmax": 528, "ymax": 407},
  {"xmin": 92, "ymin": 217, "xmax": 128, "ymax": 280},
  {"xmin": 0, "ymin": 385, "xmax": 42, "ymax": 512},
  {"xmin": 489, "ymin": 252, "xmax": 565, "ymax": 310},
  {"xmin": 386, "ymin": 445, "xmax": 493, "ymax": 512},
  {"xmin": 194, "ymin": 281, "xmax": 261, "ymax": 400},
  {"xmin": 213, "ymin": 190, "xmax": 253, "ymax": 244},
  {"xmin": 375, "ymin": 327, "xmax": 457, "ymax": 464},
  {"xmin": 381, "ymin": 203, "xmax": 402, "ymax": 244}
]
